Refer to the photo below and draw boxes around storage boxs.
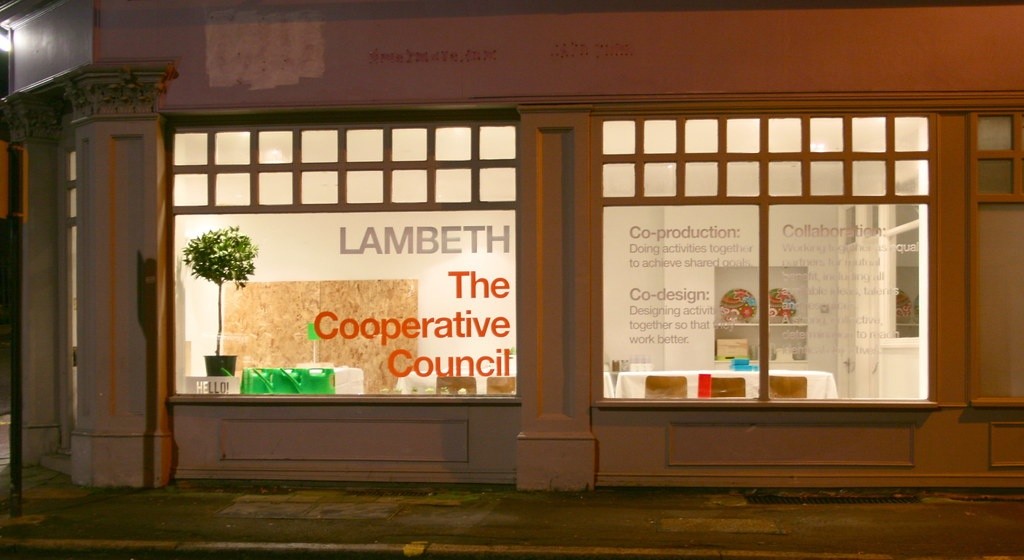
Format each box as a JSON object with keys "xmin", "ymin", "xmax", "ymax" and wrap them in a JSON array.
[{"xmin": 717, "ymin": 338, "xmax": 749, "ymax": 362}]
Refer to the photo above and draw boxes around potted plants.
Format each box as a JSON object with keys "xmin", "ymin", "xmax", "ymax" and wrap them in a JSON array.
[{"xmin": 183, "ymin": 226, "xmax": 260, "ymax": 376}]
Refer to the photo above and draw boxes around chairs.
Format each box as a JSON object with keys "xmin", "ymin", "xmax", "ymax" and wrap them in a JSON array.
[
  {"xmin": 646, "ymin": 376, "xmax": 809, "ymax": 399},
  {"xmin": 436, "ymin": 376, "xmax": 516, "ymax": 394}
]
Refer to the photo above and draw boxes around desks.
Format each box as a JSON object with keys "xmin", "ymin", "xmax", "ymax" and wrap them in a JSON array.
[
  {"xmin": 284, "ymin": 366, "xmax": 364, "ymax": 395},
  {"xmin": 184, "ymin": 375, "xmax": 242, "ymax": 395},
  {"xmin": 616, "ymin": 370, "xmax": 838, "ymax": 399}
]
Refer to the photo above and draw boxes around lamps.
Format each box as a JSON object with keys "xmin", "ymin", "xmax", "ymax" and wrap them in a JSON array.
[{"xmin": 297, "ymin": 324, "xmax": 335, "ymax": 369}]
